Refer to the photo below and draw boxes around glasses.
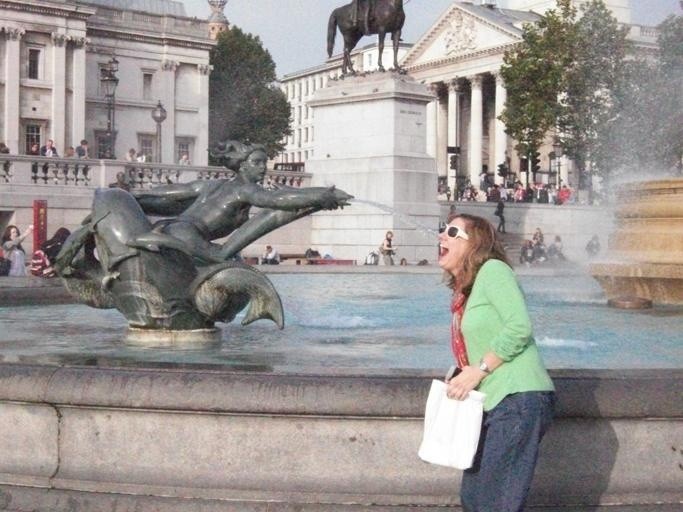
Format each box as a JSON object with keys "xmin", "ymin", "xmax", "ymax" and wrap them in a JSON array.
[{"xmin": 437, "ymin": 221, "xmax": 469, "ymax": 244}]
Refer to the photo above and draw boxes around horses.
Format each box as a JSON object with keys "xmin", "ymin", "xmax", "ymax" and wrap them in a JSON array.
[{"xmin": 327, "ymin": 0, "xmax": 405, "ymax": 75}]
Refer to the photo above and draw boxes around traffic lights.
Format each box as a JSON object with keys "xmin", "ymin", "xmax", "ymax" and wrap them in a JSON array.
[
  {"xmin": 530, "ymin": 152, "xmax": 541, "ymax": 173},
  {"xmin": 449, "ymin": 155, "xmax": 455, "ymax": 168},
  {"xmin": 497, "ymin": 164, "xmax": 503, "ymax": 176}
]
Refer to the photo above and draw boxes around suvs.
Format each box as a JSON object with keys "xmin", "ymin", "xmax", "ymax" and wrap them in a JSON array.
[{"xmin": 271, "ymin": 163, "xmax": 304, "ymax": 187}]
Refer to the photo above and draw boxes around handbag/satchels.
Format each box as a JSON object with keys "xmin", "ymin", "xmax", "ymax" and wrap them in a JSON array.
[{"xmin": 416, "ymin": 376, "xmax": 487, "ymax": 472}]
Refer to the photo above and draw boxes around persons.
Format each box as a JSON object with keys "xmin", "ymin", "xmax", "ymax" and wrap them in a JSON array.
[
  {"xmin": 126, "ymin": 138, "xmax": 345, "ymax": 259},
  {"xmin": 126, "ymin": 145, "xmax": 136, "ymax": 164},
  {"xmin": 400, "ymin": 258, "xmax": 407, "ymax": 266},
  {"xmin": 135, "ymin": 149, "xmax": 148, "ymax": 165},
  {"xmin": 426, "ymin": 172, "xmax": 602, "ymax": 267},
  {"xmin": 432, "ymin": 212, "xmax": 556, "ymax": 512},
  {"xmin": 380, "ymin": 231, "xmax": 396, "ymax": 265},
  {"xmin": 263, "ymin": 245, "xmax": 281, "ymax": 265},
  {"xmin": 27, "ymin": 137, "xmax": 91, "ymax": 159},
  {"xmin": 178, "ymin": 151, "xmax": 192, "ymax": 167},
  {"xmin": 1, "ymin": 141, "xmax": 11, "ymax": 154},
  {"xmin": 39, "ymin": 227, "xmax": 71, "ymax": 276},
  {"xmin": 2, "ymin": 224, "xmax": 36, "ymax": 280}
]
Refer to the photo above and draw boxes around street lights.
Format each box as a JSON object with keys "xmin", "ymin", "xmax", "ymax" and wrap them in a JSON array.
[
  {"xmin": 108, "ymin": 55, "xmax": 119, "ymax": 157},
  {"xmin": 552, "ymin": 142, "xmax": 563, "ymax": 190},
  {"xmin": 149, "ymin": 101, "xmax": 167, "ymax": 163},
  {"xmin": 452, "ymin": 75, "xmax": 462, "ymax": 147},
  {"xmin": 98, "ymin": 70, "xmax": 119, "ymax": 159}
]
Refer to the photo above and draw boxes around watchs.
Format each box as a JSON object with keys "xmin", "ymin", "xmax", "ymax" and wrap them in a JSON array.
[{"xmin": 478, "ymin": 358, "xmax": 490, "ymax": 373}]
